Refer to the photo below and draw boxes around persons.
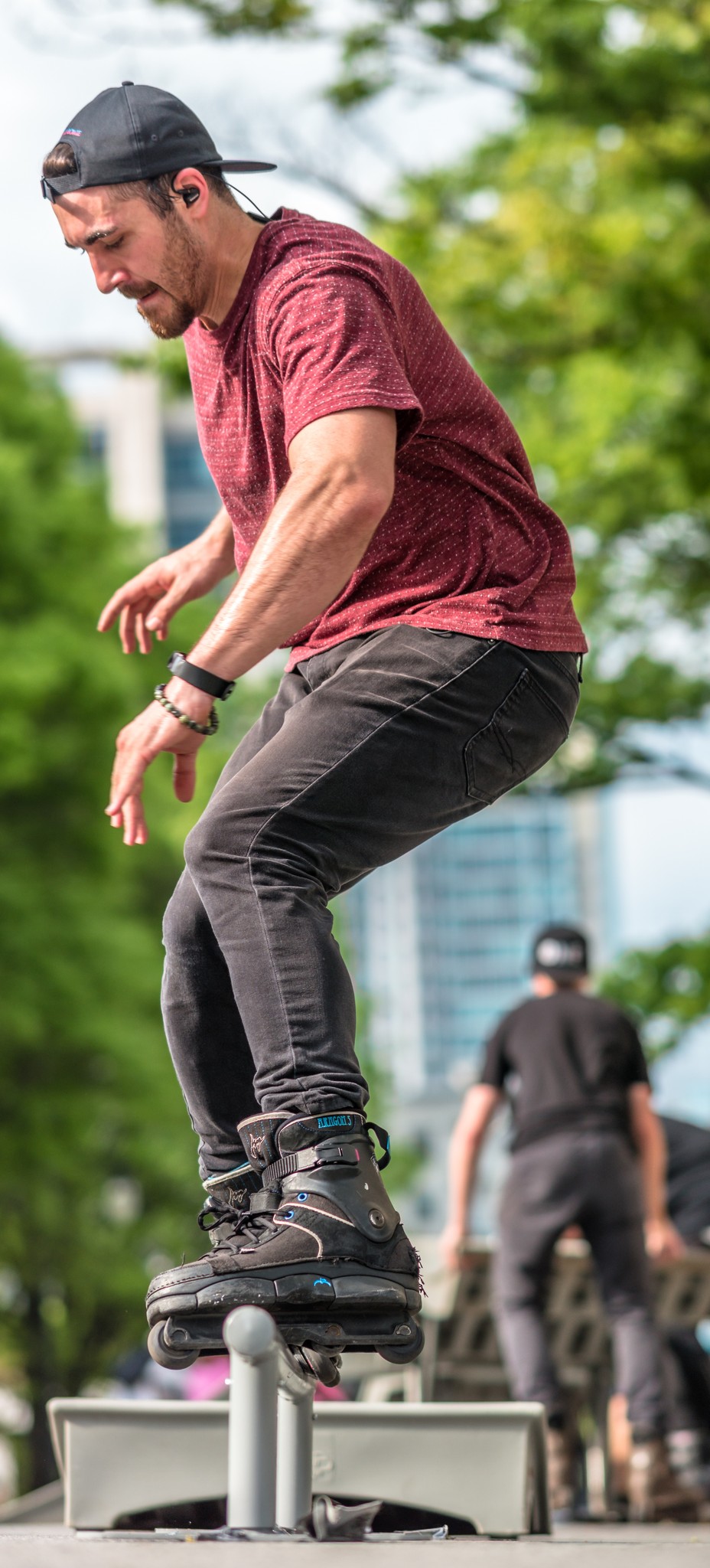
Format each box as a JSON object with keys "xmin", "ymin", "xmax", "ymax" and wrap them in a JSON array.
[
  {"xmin": 42, "ymin": 87, "xmax": 588, "ymax": 1385},
  {"xmin": 559, "ymin": 1111, "xmax": 710, "ymax": 1519},
  {"xmin": 446, "ymin": 922, "xmax": 700, "ymax": 1524}
]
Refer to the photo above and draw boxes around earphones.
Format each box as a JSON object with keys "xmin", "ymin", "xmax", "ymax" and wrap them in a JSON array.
[{"xmin": 178, "ymin": 186, "xmax": 200, "ymax": 202}]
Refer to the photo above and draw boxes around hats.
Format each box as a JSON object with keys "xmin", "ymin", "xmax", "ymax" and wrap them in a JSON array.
[
  {"xmin": 531, "ymin": 925, "xmax": 588, "ymax": 979},
  {"xmin": 42, "ymin": 80, "xmax": 277, "ymax": 204}
]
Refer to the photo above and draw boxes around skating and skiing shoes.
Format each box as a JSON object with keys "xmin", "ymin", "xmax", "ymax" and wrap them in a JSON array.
[
  {"xmin": 146, "ymin": 1111, "xmax": 426, "ymax": 1372},
  {"xmin": 197, "ymin": 1165, "xmax": 342, "ymax": 1389}
]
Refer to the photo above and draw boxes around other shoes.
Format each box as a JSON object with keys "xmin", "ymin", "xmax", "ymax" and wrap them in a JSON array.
[
  {"xmin": 626, "ymin": 1438, "xmax": 710, "ymax": 1524},
  {"xmin": 546, "ymin": 1408, "xmax": 587, "ymax": 1521}
]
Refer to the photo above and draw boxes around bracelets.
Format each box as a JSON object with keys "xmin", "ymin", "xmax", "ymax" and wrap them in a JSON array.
[
  {"xmin": 167, "ymin": 651, "xmax": 236, "ymax": 701},
  {"xmin": 154, "ymin": 684, "xmax": 220, "ymax": 736}
]
